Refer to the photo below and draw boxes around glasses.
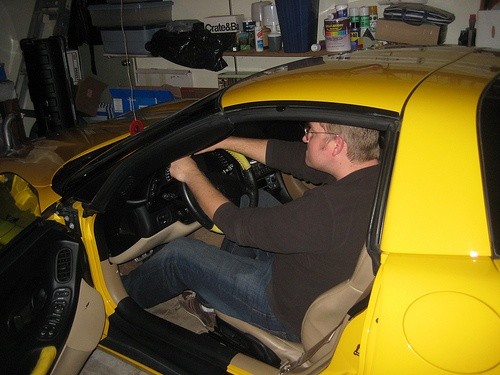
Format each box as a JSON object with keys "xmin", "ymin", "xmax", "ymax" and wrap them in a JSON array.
[{"xmin": 304, "ymin": 127, "xmax": 345, "ymax": 143}]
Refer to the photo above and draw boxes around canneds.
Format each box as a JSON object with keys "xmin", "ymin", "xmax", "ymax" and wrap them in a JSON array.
[{"xmin": 324, "ymin": 17, "xmax": 352, "ymax": 52}]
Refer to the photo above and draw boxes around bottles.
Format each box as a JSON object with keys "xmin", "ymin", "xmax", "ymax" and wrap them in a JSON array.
[
  {"xmin": 254, "ymin": 22, "xmax": 264, "ymax": 52},
  {"xmin": 349, "ymin": 5, "xmax": 377, "ymax": 50},
  {"xmin": 327, "ymin": 13, "xmax": 338, "ymax": 20}
]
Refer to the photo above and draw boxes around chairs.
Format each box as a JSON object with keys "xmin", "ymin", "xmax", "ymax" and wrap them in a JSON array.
[{"xmin": 214, "ymin": 244, "xmax": 378, "ymax": 373}]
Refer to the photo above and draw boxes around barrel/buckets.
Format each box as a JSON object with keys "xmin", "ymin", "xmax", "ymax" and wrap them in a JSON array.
[{"xmin": 324, "ymin": 18, "xmax": 351, "ymax": 52}]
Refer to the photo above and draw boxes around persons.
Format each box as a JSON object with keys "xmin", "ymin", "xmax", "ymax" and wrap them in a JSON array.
[{"xmin": 119, "ymin": 121, "xmax": 381, "ymax": 344}]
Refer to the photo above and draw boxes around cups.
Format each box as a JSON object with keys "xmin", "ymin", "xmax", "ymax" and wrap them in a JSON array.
[
  {"xmin": 243, "ymin": 20, "xmax": 255, "ymax": 47},
  {"xmin": 336, "ymin": 4, "xmax": 347, "ymax": 18},
  {"xmin": 268, "ymin": 34, "xmax": 282, "ymax": 51}
]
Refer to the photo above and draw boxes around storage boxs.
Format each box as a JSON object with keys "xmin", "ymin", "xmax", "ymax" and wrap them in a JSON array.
[
  {"xmin": 373, "ymin": 16, "xmax": 450, "ymax": 45},
  {"xmin": 88, "ymin": 1, "xmax": 245, "ymax": 54}
]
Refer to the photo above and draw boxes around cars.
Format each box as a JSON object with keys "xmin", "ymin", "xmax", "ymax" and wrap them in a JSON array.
[{"xmin": 1, "ymin": 44, "xmax": 500, "ymax": 375}]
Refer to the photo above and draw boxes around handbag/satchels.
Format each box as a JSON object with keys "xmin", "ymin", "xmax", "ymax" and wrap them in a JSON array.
[{"xmin": 384, "ymin": 2, "xmax": 455, "ymax": 27}]
